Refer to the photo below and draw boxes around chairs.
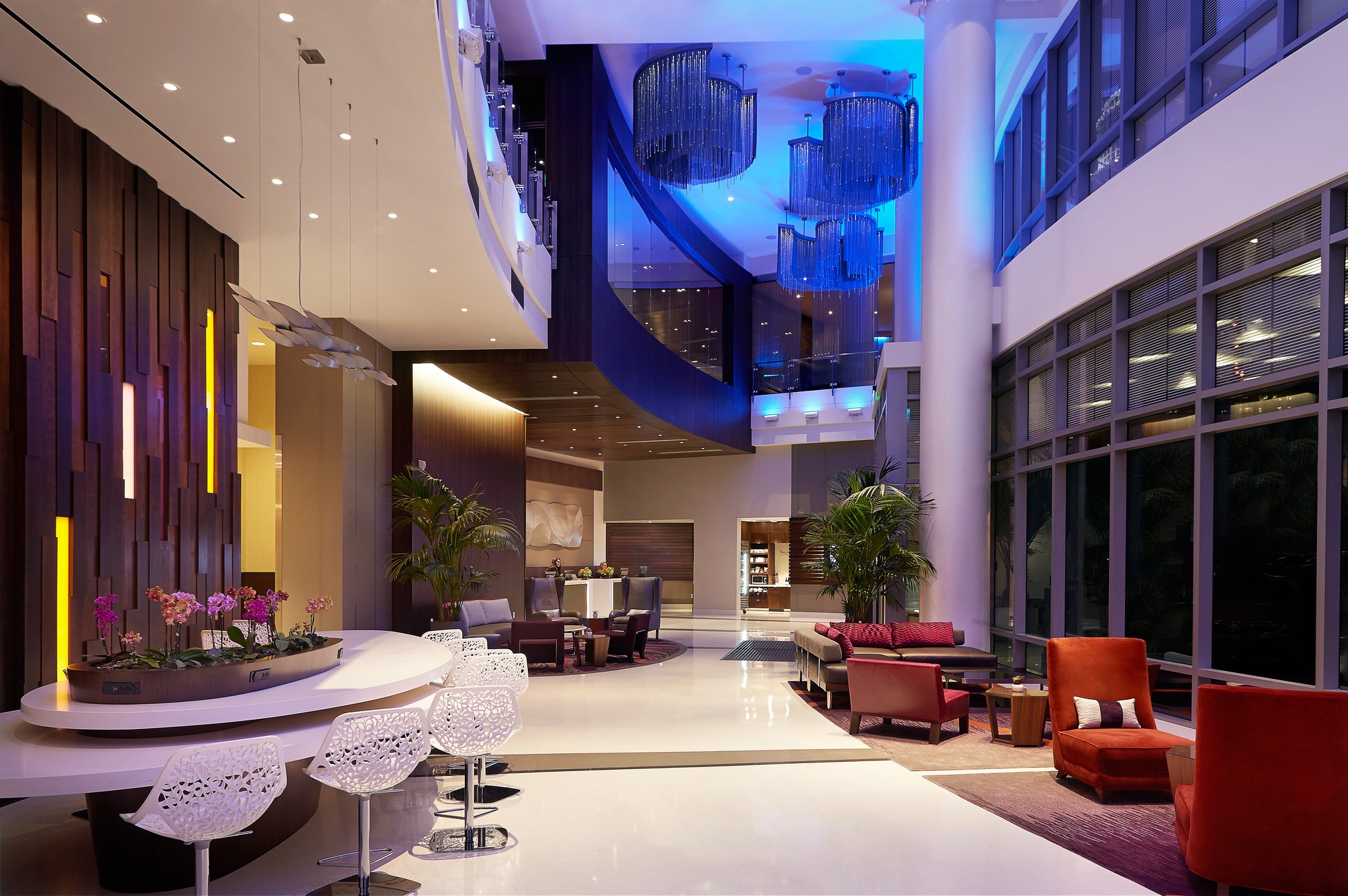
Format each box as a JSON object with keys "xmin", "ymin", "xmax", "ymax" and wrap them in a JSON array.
[
  {"xmin": 1174, "ymin": 683, "xmax": 1348, "ymax": 896},
  {"xmin": 1046, "ymin": 637, "xmax": 1196, "ymax": 804},
  {"xmin": 510, "ymin": 575, "xmax": 664, "ymax": 673}
]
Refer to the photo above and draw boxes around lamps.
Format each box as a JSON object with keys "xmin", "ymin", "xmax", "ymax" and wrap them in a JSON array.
[{"xmin": 632, "ymin": 42, "xmax": 921, "ymax": 301}]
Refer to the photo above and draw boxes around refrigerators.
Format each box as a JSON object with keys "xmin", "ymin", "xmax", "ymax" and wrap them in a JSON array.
[{"xmin": 740, "ymin": 541, "xmax": 749, "ymax": 613}]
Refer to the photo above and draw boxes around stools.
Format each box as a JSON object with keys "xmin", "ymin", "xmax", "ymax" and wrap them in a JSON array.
[{"xmin": 122, "ymin": 626, "xmax": 530, "ymax": 896}]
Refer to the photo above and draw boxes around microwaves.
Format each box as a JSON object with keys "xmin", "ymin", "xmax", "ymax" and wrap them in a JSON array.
[{"xmin": 752, "ymin": 575, "xmax": 767, "ymax": 584}]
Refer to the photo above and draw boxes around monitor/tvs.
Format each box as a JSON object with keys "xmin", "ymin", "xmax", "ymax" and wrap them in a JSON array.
[
  {"xmin": 619, "ymin": 568, "xmax": 629, "ymax": 576},
  {"xmin": 638, "ymin": 566, "xmax": 647, "ymax": 577},
  {"xmin": 562, "ymin": 570, "xmax": 574, "ymax": 580},
  {"xmin": 543, "ymin": 571, "xmax": 556, "ymax": 578}
]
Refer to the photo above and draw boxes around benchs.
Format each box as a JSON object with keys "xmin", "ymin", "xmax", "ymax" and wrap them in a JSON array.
[
  {"xmin": 440, "ymin": 598, "xmax": 513, "ymax": 649},
  {"xmin": 793, "ymin": 621, "xmax": 999, "ymax": 708},
  {"xmin": 846, "ymin": 657, "xmax": 970, "ymax": 746}
]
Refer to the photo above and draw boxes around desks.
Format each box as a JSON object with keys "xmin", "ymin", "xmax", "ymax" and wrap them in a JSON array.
[{"xmin": 985, "ymin": 687, "xmax": 1049, "ymax": 749}]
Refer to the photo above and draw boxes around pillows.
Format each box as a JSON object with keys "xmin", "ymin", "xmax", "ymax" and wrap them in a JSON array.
[
  {"xmin": 814, "ymin": 622, "xmax": 854, "ymax": 659},
  {"xmin": 1073, "ymin": 696, "xmax": 1141, "ymax": 729},
  {"xmin": 829, "ymin": 621, "xmax": 892, "ymax": 647},
  {"xmin": 890, "ymin": 621, "xmax": 956, "ymax": 649}
]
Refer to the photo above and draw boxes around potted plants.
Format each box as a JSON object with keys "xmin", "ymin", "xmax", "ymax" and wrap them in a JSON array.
[
  {"xmin": 578, "ymin": 566, "xmax": 614, "ymax": 580},
  {"xmin": 1012, "ymin": 675, "xmax": 1024, "ymax": 692}
]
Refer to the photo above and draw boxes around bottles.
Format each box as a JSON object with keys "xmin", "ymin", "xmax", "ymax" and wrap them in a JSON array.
[{"xmin": 740, "ymin": 552, "xmax": 745, "ymax": 596}]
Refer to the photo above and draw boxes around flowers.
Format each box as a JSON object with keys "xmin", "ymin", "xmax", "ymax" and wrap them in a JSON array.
[{"xmin": 90, "ymin": 588, "xmax": 335, "ymax": 670}]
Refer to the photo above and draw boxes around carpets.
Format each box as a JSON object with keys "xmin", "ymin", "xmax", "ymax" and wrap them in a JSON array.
[
  {"xmin": 505, "ymin": 635, "xmax": 687, "ymax": 677},
  {"xmin": 926, "ymin": 772, "xmax": 1250, "ymax": 896},
  {"xmin": 720, "ymin": 640, "xmax": 794, "ymax": 662},
  {"xmin": 786, "ymin": 680, "xmax": 1058, "ymax": 772}
]
